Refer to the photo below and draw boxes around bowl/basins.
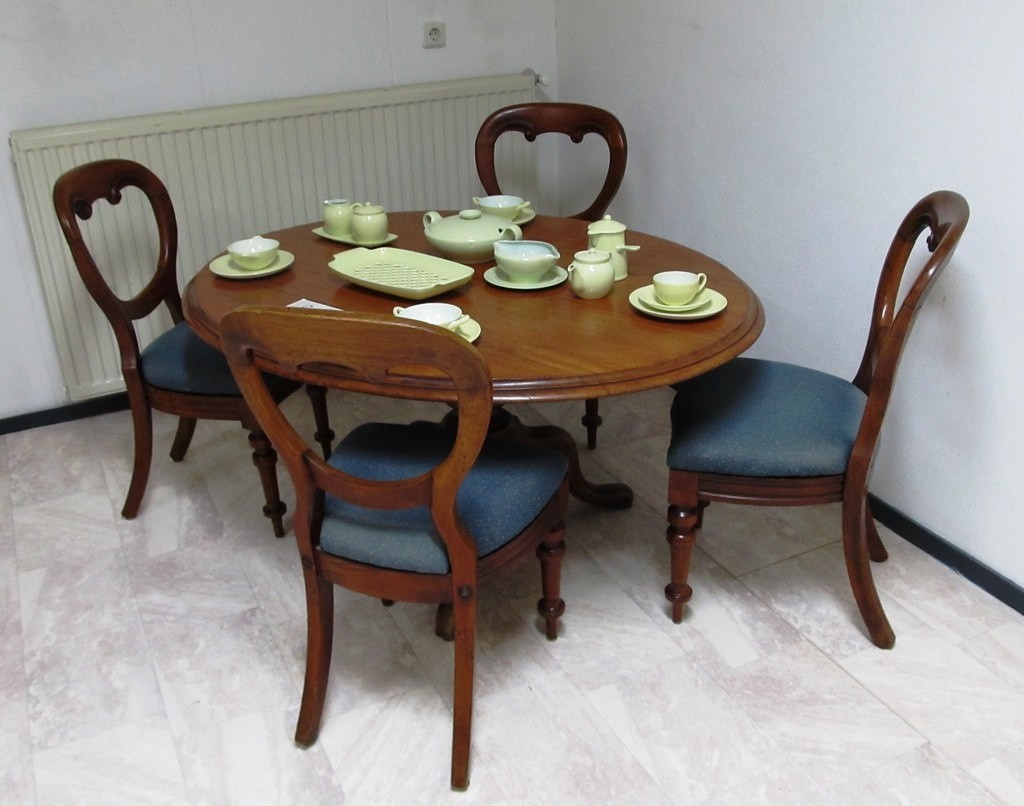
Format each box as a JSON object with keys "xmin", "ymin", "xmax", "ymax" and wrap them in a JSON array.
[
  {"xmin": 393, "ymin": 302, "xmax": 470, "ymax": 333},
  {"xmin": 226, "ymin": 235, "xmax": 280, "ymax": 270},
  {"xmin": 422, "ymin": 209, "xmax": 523, "ymax": 264},
  {"xmin": 472, "ymin": 195, "xmax": 530, "ymax": 221},
  {"xmin": 492, "ymin": 240, "xmax": 560, "ymax": 283}
]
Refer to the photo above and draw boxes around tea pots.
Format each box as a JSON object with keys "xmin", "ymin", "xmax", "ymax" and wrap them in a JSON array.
[
  {"xmin": 567, "ymin": 248, "xmax": 615, "ymax": 300},
  {"xmin": 587, "ymin": 215, "xmax": 640, "ymax": 282}
]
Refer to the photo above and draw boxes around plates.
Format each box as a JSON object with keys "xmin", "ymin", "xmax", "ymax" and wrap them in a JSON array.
[
  {"xmin": 312, "ymin": 224, "xmax": 398, "ymax": 247},
  {"xmin": 456, "ymin": 315, "xmax": 481, "ymax": 343},
  {"xmin": 638, "ymin": 287, "xmax": 712, "ymax": 312},
  {"xmin": 209, "ymin": 249, "xmax": 294, "ymax": 279},
  {"xmin": 327, "ymin": 247, "xmax": 475, "ymax": 299},
  {"xmin": 484, "ymin": 265, "xmax": 568, "ymax": 289},
  {"xmin": 629, "ymin": 283, "xmax": 728, "ymax": 319},
  {"xmin": 512, "ymin": 208, "xmax": 535, "ymax": 224}
]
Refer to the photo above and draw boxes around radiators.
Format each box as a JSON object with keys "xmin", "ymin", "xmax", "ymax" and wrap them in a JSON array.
[{"xmin": 9, "ymin": 69, "xmax": 536, "ymax": 402}]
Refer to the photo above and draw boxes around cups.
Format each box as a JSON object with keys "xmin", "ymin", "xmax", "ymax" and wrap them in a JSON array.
[
  {"xmin": 652, "ymin": 271, "xmax": 707, "ymax": 306},
  {"xmin": 351, "ymin": 202, "xmax": 388, "ymax": 242}
]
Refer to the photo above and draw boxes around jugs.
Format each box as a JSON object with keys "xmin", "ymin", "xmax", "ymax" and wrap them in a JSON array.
[{"xmin": 322, "ymin": 199, "xmax": 363, "ymax": 235}]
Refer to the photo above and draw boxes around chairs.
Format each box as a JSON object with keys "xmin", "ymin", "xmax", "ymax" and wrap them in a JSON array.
[
  {"xmin": 474, "ymin": 102, "xmax": 627, "ymax": 449},
  {"xmin": 664, "ymin": 191, "xmax": 969, "ymax": 649},
  {"xmin": 53, "ymin": 159, "xmax": 335, "ymax": 538},
  {"xmin": 221, "ymin": 303, "xmax": 572, "ymax": 791}
]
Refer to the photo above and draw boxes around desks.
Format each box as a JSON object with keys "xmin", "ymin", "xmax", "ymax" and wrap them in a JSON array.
[{"xmin": 181, "ymin": 211, "xmax": 766, "ymax": 641}]
